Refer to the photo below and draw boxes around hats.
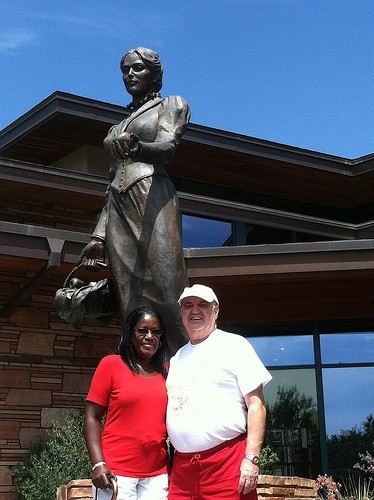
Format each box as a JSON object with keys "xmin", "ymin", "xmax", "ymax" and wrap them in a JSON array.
[{"xmin": 177, "ymin": 284, "xmax": 219, "ymax": 308}]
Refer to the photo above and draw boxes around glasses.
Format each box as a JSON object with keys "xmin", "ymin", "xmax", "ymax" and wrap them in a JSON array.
[{"xmin": 132, "ymin": 328, "xmax": 164, "ymax": 337}]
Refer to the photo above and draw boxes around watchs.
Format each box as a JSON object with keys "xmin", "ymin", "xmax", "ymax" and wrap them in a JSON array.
[{"xmin": 245, "ymin": 454, "xmax": 261, "ymax": 466}]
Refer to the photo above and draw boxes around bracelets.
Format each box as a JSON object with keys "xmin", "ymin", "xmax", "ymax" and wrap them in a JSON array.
[{"xmin": 92, "ymin": 460, "xmax": 106, "ymax": 471}]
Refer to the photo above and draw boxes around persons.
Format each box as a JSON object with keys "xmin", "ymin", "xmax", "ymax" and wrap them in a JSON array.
[
  {"xmin": 165, "ymin": 284, "xmax": 273, "ymax": 500},
  {"xmin": 79, "ymin": 304, "xmax": 170, "ymax": 500},
  {"xmin": 75, "ymin": 47, "xmax": 191, "ymax": 362}
]
